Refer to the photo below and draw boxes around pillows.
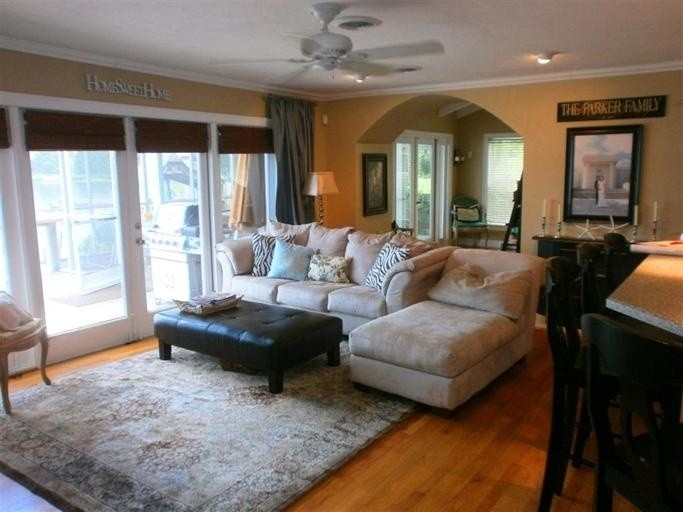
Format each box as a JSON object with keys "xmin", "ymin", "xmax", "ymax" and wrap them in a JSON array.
[
  {"xmin": 362, "ymin": 242, "xmax": 412, "ymax": 292},
  {"xmin": 264, "ymin": 238, "xmax": 314, "ymax": 282},
  {"xmin": 306, "ymin": 256, "xmax": 351, "ymax": 285},
  {"xmin": 427, "ymin": 263, "xmax": 533, "ymax": 318},
  {"xmin": 345, "ymin": 231, "xmax": 393, "ymax": 285},
  {"xmin": 306, "ymin": 224, "xmax": 353, "ymax": 258},
  {"xmin": 248, "ymin": 230, "xmax": 296, "ymax": 277},
  {"xmin": 388, "ymin": 231, "xmax": 434, "ymax": 258}
]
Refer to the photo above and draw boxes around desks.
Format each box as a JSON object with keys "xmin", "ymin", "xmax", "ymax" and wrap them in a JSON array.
[{"xmin": 0, "ymin": 316, "xmax": 52, "ymax": 413}]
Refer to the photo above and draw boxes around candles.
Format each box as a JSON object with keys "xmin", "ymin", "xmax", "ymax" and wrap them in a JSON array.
[
  {"xmin": 654, "ymin": 202, "xmax": 658, "ymax": 221},
  {"xmin": 543, "ymin": 200, "xmax": 546, "ymax": 217},
  {"xmin": 557, "ymin": 205, "xmax": 561, "ymax": 221},
  {"xmin": 634, "ymin": 205, "xmax": 638, "ymax": 225}
]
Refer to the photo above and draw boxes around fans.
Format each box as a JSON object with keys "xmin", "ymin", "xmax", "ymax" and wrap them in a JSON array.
[{"xmin": 208, "ymin": 3, "xmax": 444, "ymax": 85}]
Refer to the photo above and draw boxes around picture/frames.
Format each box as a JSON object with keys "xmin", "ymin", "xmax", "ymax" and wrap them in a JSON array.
[
  {"xmin": 563, "ymin": 124, "xmax": 641, "ymax": 224},
  {"xmin": 361, "ymin": 152, "xmax": 388, "ymax": 218}
]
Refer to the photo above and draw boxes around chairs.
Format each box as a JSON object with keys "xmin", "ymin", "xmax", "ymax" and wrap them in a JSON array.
[
  {"xmin": 573, "ymin": 242, "xmax": 660, "ymax": 466},
  {"xmin": 604, "ymin": 234, "xmax": 631, "ymax": 309},
  {"xmin": 450, "ymin": 196, "xmax": 490, "ymax": 250},
  {"xmin": 530, "ymin": 258, "xmax": 666, "ymax": 512},
  {"xmin": 579, "ymin": 313, "xmax": 681, "ymax": 511}
]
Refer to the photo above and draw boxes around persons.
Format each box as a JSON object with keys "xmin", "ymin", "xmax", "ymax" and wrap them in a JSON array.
[{"xmin": 594, "ymin": 175, "xmax": 606, "ymax": 207}]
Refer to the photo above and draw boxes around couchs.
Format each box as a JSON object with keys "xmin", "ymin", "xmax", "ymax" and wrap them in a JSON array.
[
  {"xmin": 347, "ymin": 248, "xmax": 545, "ymax": 419},
  {"xmin": 214, "ymin": 222, "xmax": 457, "ymax": 336}
]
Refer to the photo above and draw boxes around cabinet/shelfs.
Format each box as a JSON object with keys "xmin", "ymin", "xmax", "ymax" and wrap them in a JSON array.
[{"xmin": 531, "ymin": 233, "xmax": 632, "ymax": 348}]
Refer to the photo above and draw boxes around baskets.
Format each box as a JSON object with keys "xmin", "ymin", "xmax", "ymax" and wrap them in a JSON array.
[{"xmin": 172, "ymin": 291, "xmax": 245, "ymax": 316}]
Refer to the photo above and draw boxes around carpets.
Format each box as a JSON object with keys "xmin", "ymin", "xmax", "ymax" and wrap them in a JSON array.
[{"xmin": 0, "ymin": 336, "xmax": 424, "ymax": 512}]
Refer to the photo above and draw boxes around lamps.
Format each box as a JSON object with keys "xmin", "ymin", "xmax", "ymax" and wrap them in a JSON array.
[{"xmin": 302, "ymin": 170, "xmax": 340, "ymax": 225}]
